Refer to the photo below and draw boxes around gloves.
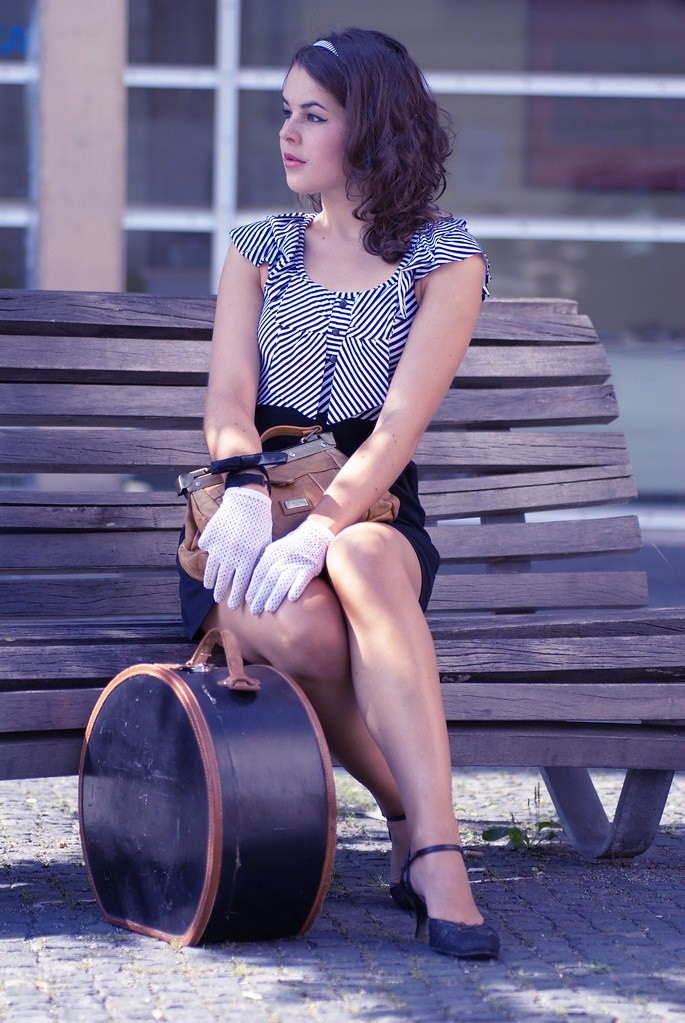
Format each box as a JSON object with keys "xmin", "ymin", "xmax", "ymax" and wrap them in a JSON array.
[
  {"xmin": 198, "ymin": 487, "xmax": 272, "ymax": 612},
  {"xmin": 245, "ymin": 520, "xmax": 335, "ymax": 616}
]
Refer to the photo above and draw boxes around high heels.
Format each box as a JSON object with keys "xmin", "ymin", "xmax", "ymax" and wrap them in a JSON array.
[
  {"xmin": 390, "ymin": 878, "xmax": 414, "ymax": 910},
  {"xmin": 400, "ymin": 845, "xmax": 501, "ymax": 960}
]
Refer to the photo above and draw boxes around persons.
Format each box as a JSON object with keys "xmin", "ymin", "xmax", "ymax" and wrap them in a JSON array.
[{"xmin": 176, "ymin": 25, "xmax": 502, "ymax": 960}]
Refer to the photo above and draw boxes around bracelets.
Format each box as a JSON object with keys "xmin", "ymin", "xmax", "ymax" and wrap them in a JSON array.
[{"xmin": 211, "ymin": 452, "xmax": 289, "ymax": 497}]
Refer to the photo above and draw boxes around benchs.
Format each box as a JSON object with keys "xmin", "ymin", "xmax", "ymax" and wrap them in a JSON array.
[{"xmin": 0, "ymin": 283, "xmax": 685, "ymax": 861}]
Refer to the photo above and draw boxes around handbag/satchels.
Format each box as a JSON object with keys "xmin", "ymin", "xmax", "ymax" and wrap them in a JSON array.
[{"xmin": 175, "ymin": 425, "xmax": 400, "ymax": 581}]
